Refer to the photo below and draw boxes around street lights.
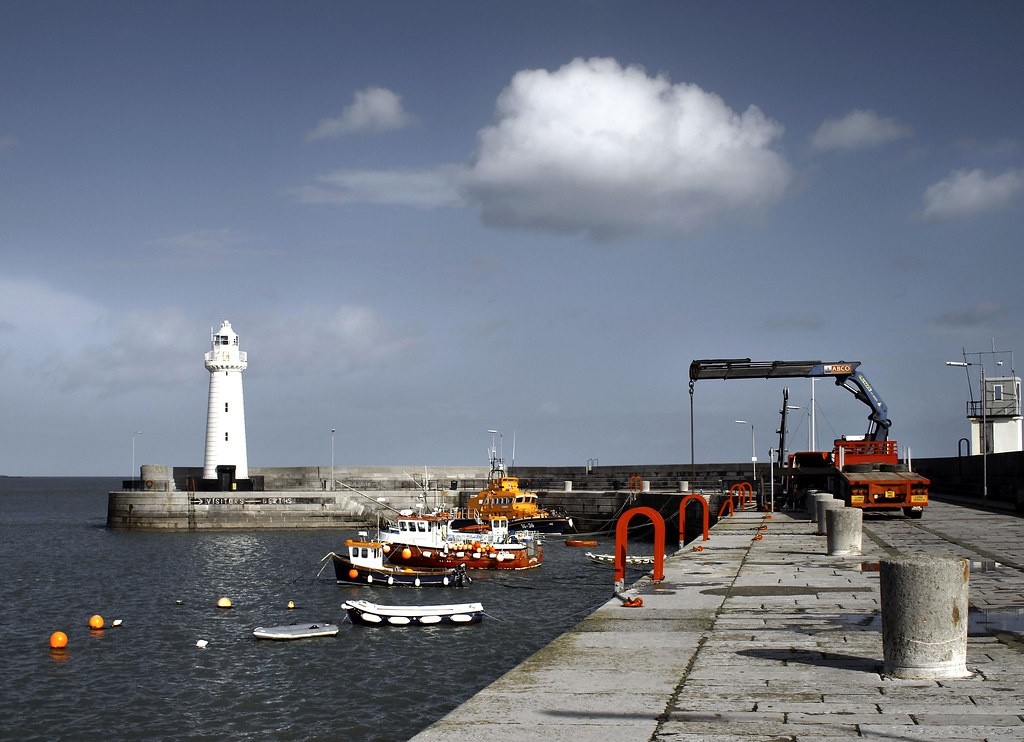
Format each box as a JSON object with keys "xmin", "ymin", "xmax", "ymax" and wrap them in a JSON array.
[
  {"xmin": 945, "ymin": 361, "xmax": 988, "ymax": 497},
  {"xmin": 734, "ymin": 420, "xmax": 756, "ymax": 482},
  {"xmin": 487, "ymin": 430, "xmax": 503, "ymax": 466}
]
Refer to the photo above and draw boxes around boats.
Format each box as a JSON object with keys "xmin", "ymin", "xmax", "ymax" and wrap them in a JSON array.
[
  {"xmin": 340, "ymin": 600, "xmax": 485, "ymax": 628},
  {"xmin": 252, "ymin": 622, "xmax": 340, "ymax": 640},
  {"xmin": 333, "ymin": 428, "xmax": 573, "ymax": 569},
  {"xmin": 331, "ymin": 531, "xmax": 474, "ymax": 589},
  {"xmin": 584, "ymin": 551, "xmax": 668, "ymax": 564},
  {"xmin": 564, "ymin": 539, "xmax": 598, "ymax": 547}
]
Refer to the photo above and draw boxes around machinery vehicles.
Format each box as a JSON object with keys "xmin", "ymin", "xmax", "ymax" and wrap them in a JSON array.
[{"xmin": 687, "ymin": 358, "xmax": 931, "ymax": 519}]
